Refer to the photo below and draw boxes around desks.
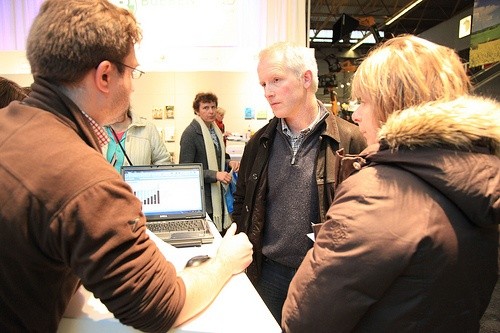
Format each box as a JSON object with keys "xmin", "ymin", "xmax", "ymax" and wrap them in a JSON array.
[{"xmin": 55, "ymin": 213, "xmax": 282, "ymax": 333}]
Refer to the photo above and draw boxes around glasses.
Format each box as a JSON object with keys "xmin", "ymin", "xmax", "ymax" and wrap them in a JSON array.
[{"xmin": 95, "ymin": 58, "xmax": 145, "ymax": 80}]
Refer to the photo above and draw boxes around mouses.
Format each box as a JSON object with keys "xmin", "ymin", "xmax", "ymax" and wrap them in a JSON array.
[{"xmin": 184, "ymin": 254, "xmax": 210, "ymax": 267}]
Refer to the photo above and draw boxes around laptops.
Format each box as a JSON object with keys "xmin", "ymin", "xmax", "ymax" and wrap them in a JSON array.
[{"xmin": 119, "ymin": 163, "xmax": 214, "ymax": 248}]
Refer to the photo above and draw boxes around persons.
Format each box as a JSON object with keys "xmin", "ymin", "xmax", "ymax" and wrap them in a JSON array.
[
  {"xmin": 101, "ymin": 108, "xmax": 173, "ymax": 175},
  {"xmin": 0, "ymin": 0, "xmax": 253, "ymax": 333},
  {"xmin": 282, "ymin": 33, "xmax": 500, "ymax": 333},
  {"xmin": 179, "ymin": 93, "xmax": 241, "ymax": 238},
  {"xmin": 232, "ymin": 42, "xmax": 368, "ymax": 328},
  {"xmin": 0, "ymin": 77, "xmax": 91, "ymax": 319}
]
